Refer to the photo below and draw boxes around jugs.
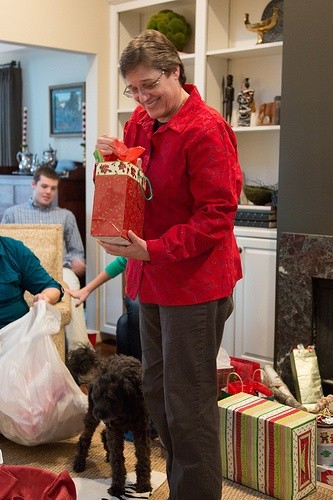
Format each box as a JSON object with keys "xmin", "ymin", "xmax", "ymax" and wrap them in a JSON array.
[
  {"xmin": 17, "ymin": 148, "xmax": 38, "ymax": 172},
  {"xmin": 41, "ymin": 144, "xmax": 58, "ymax": 169}
]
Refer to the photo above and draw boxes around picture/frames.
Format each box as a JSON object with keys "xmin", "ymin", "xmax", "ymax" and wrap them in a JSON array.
[{"xmin": 49, "ymin": 82, "xmax": 85, "ymax": 138}]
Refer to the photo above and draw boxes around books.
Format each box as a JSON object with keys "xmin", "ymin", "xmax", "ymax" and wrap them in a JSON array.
[{"xmin": 235, "ymin": 204, "xmax": 277, "ymax": 228}]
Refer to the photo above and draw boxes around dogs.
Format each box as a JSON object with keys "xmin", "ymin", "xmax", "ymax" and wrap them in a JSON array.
[{"xmin": 67, "ymin": 347, "xmax": 154, "ymax": 497}]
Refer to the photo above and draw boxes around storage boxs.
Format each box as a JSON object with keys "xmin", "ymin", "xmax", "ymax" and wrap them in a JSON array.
[{"xmin": 218, "ymin": 392, "xmax": 317, "ymax": 500}]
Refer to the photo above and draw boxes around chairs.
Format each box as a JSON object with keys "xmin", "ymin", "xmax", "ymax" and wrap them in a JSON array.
[{"xmin": 0, "ymin": 224, "xmax": 71, "ymax": 369}]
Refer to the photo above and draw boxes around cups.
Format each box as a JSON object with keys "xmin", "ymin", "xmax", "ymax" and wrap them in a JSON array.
[{"xmin": 88, "ymin": 332, "xmax": 96, "ymax": 348}]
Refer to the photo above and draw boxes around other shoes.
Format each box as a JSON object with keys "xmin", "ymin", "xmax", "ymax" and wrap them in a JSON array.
[{"xmin": 69, "ymin": 349, "xmax": 95, "ymax": 374}]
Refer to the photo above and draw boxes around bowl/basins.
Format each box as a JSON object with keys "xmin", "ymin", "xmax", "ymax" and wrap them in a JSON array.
[{"xmin": 244, "ymin": 185, "xmax": 273, "ymax": 205}]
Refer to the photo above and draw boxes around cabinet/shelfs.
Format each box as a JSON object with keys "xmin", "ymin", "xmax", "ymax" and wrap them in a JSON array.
[
  {"xmin": 0, "ymin": 174, "xmax": 87, "ymax": 230},
  {"xmin": 110, "ymin": 0, "xmax": 285, "ymax": 241},
  {"xmin": 101, "ymin": 234, "xmax": 278, "ymax": 370}
]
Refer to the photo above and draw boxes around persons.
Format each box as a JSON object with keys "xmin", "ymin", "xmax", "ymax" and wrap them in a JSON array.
[
  {"xmin": 61, "ymin": 251, "xmax": 141, "ymax": 361},
  {"xmin": 93, "ymin": 28, "xmax": 241, "ymax": 500},
  {"xmin": 1, "ymin": 167, "xmax": 96, "ymax": 358},
  {"xmin": 224, "ymin": 75, "xmax": 234, "ymax": 127},
  {"xmin": 0, "ymin": 237, "xmax": 63, "ymax": 330},
  {"xmin": 235, "ymin": 77, "xmax": 254, "ymax": 127}
]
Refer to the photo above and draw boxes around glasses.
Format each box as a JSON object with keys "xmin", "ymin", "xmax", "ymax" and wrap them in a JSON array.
[{"xmin": 123, "ymin": 69, "xmax": 166, "ymax": 99}]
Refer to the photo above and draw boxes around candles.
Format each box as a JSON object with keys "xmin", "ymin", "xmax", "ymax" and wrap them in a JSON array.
[
  {"xmin": 82, "ymin": 102, "xmax": 85, "ymax": 138},
  {"xmin": 23, "ymin": 107, "xmax": 28, "ymax": 144}
]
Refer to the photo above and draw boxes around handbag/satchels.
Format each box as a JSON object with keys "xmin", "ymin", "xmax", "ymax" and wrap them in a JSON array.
[
  {"xmin": 221, "ymin": 371, "xmax": 271, "ymax": 399},
  {"xmin": 0, "ymin": 300, "xmax": 89, "ymax": 445},
  {"xmin": 90, "ymin": 160, "xmax": 153, "ymax": 247},
  {"xmin": 289, "ymin": 349, "xmax": 324, "ymax": 406}
]
